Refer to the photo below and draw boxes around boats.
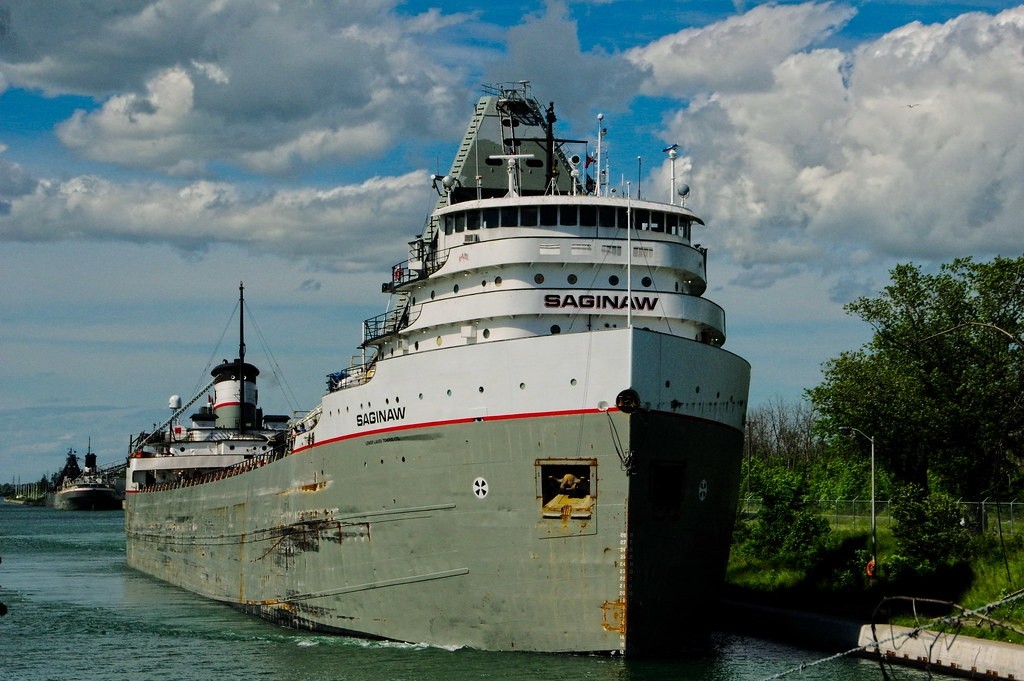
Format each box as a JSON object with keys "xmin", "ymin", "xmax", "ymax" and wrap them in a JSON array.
[
  {"xmin": 122, "ymin": 79, "xmax": 755, "ymax": 657},
  {"xmin": 4, "ymin": 483, "xmax": 49, "ymax": 506},
  {"xmin": 53, "ymin": 436, "xmax": 117, "ymax": 510}
]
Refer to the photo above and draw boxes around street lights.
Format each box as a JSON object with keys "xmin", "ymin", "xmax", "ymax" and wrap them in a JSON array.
[{"xmin": 839, "ymin": 425, "xmax": 876, "ymax": 579}]
